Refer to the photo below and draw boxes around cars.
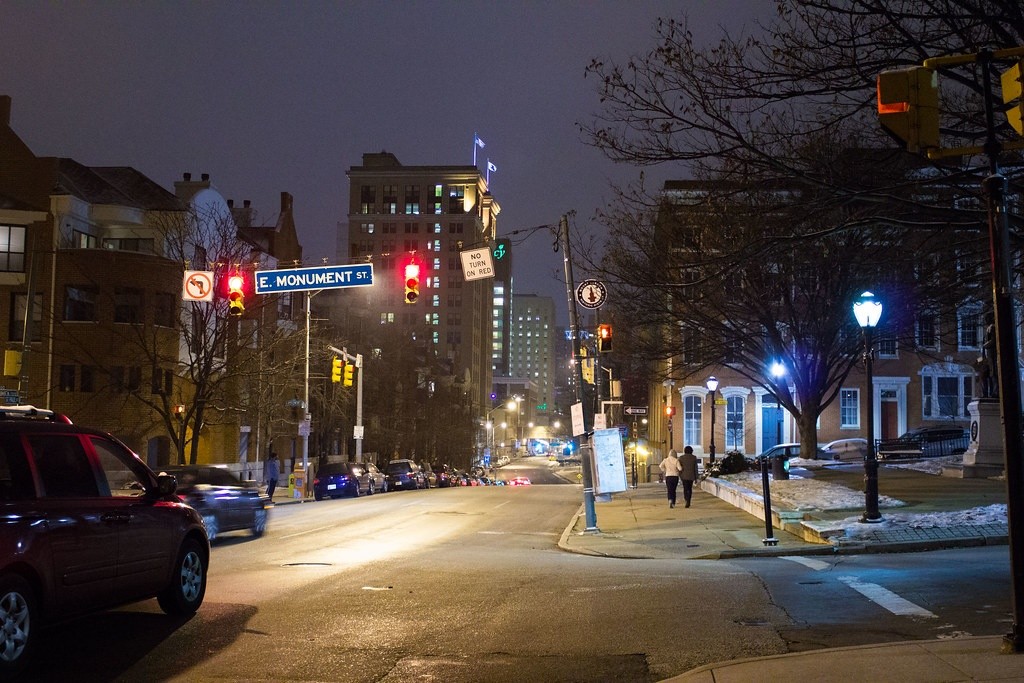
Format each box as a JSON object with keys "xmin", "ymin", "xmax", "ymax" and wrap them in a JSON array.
[
  {"xmin": 154, "ymin": 462, "xmax": 269, "ymax": 544},
  {"xmin": 0, "ymin": 403, "xmax": 211, "ymax": 683},
  {"xmin": 454, "ymin": 469, "xmax": 530, "ymax": 487},
  {"xmin": 753, "ymin": 442, "xmax": 829, "ymax": 471},
  {"xmin": 899, "ymin": 425, "xmax": 968, "ymax": 459},
  {"xmin": 358, "ymin": 455, "xmax": 452, "ymax": 489},
  {"xmin": 315, "ymin": 459, "xmax": 372, "ymax": 501},
  {"xmin": 823, "ymin": 439, "xmax": 874, "ymax": 464}
]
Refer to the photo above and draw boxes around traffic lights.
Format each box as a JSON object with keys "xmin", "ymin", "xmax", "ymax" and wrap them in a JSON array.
[
  {"xmin": 493, "ymin": 237, "xmax": 511, "ymax": 279},
  {"xmin": 665, "ymin": 406, "xmax": 676, "ymax": 415},
  {"xmin": 874, "ymin": 65, "xmax": 941, "ymax": 152},
  {"xmin": 344, "ymin": 363, "xmax": 353, "ymax": 388},
  {"xmin": 331, "ymin": 358, "xmax": 343, "ymax": 383},
  {"xmin": 597, "ymin": 325, "xmax": 613, "ymax": 352},
  {"xmin": 228, "ymin": 274, "xmax": 243, "ymax": 317},
  {"xmin": 404, "ymin": 262, "xmax": 420, "ymax": 304}
]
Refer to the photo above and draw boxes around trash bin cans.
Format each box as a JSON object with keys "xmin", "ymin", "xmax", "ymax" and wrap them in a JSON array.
[
  {"xmin": 294, "ymin": 462, "xmax": 314, "ymax": 496},
  {"xmin": 772, "ymin": 454, "xmax": 790, "ymax": 479},
  {"xmin": 587, "ymin": 427, "xmax": 628, "ymax": 495}
]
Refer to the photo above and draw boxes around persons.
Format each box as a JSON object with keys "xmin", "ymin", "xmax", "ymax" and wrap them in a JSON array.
[
  {"xmin": 265, "ymin": 452, "xmax": 280, "ymax": 505},
  {"xmin": 679, "ymin": 446, "xmax": 698, "ymax": 508},
  {"xmin": 658, "ymin": 449, "xmax": 682, "ymax": 508}
]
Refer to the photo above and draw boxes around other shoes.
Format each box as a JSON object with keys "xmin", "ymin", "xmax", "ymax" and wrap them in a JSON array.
[
  {"xmin": 685, "ymin": 499, "xmax": 690, "ymax": 508},
  {"xmin": 669, "ymin": 500, "xmax": 675, "ymax": 508}
]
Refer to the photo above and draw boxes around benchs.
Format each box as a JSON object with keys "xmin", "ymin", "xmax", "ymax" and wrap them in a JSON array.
[{"xmin": 879, "ymin": 443, "xmax": 924, "ymax": 460}]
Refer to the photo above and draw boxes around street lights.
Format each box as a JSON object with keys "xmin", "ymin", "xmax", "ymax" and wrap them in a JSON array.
[
  {"xmin": 485, "ymin": 400, "xmax": 514, "ymax": 459},
  {"xmin": 768, "ymin": 355, "xmax": 789, "ymax": 459},
  {"xmin": 852, "ymin": 291, "xmax": 885, "ymax": 522},
  {"xmin": 491, "ymin": 421, "xmax": 507, "ymax": 461},
  {"xmin": 707, "ymin": 374, "xmax": 721, "ymax": 469}
]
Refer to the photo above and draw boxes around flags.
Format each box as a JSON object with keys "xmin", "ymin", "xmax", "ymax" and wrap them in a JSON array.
[
  {"xmin": 488, "ymin": 161, "xmax": 497, "ymax": 172},
  {"xmin": 475, "ymin": 134, "xmax": 486, "ymax": 149}
]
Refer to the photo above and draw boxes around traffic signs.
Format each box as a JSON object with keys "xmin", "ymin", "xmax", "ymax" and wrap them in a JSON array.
[{"xmin": 623, "ymin": 406, "xmax": 648, "ymax": 416}]
